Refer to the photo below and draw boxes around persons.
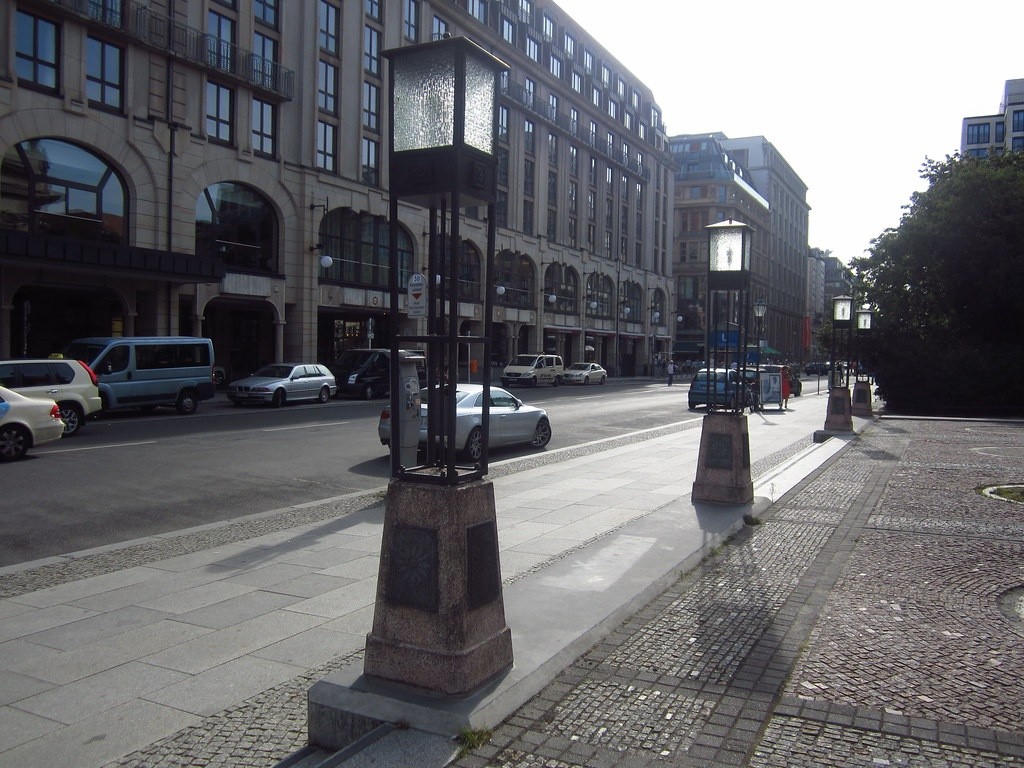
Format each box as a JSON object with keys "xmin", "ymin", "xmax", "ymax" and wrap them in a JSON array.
[
  {"xmin": 667, "ymin": 359, "xmax": 675, "ymax": 387},
  {"xmin": 835, "ymin": 361, "xmax": 845, "ymax": 384},
  {"xmin": 435, "ymin": 355, "xmax": 449, "ymax": 385},
  {"xmin": 779, "ymin": 366, "xmax": 790, "ymax": 413}
]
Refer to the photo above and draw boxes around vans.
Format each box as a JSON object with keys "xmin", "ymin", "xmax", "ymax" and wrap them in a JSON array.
[{"xmin": 499, "ymin": 352, "xmax": 565, "ymax": 389}]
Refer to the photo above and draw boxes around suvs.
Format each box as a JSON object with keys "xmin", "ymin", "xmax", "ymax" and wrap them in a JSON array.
[
  {"xmin": 63, "ymin": 336, "xmax": 216, "ymax": 416},
  {"xmin": 0, "ymin": 357, "xmax": 102, "ymax": 436}
]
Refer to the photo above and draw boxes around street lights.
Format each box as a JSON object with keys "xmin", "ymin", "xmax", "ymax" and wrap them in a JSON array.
[
  {"xmin": 356, "ymin": 30, "xmax": 519, "ymax": 690},
  {"xmin": 852, "ymin": 304, "xmax": 876, "ymax": 417},
  {"xmin": 817, "ymin": 290, "xmax": 856, "ymax": 434},
  {"xmin": 691, "ymin": 214, "xmax": 755, "ymax": 505}
]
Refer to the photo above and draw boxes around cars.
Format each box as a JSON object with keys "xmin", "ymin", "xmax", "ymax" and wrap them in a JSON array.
[
  {"xmin": 755, "ymin": 363, "xmax": 801, "ymax": 396},
  {"xmin": 0, "ymin": 386, "xmax": 64, "ymax": 461},
  {"xmin": 561, "ymin": 362, "xmax": 610, "ymax": 387},
  {"xmin": 378, "ymin": 381, "xmax": 552, "ymax": 459},
  {"xmin": 733, "ymin": 365, "xmax": 766, "ymax": 397},
  {"xmin": 331, "ymin": 349, "xmax": 428, "ymax": 398},
  {"xmin": 686, "ymin": 367, "xmax": 747, "ymax": 409},
  {"xmin": 805, "ymin": 361, "xmax": 856, "ymax": 377},
  {"xmin": 228, "ymin": 362, "xmax": 338, "ymax": 409}
]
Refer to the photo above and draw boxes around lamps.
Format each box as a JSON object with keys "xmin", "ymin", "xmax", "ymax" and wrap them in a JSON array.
[
  {"xmin": 672, "ymin": 310, "xmax": 683, "ymax": 323},
  {"xmin": 421, "ymin": 265, "xmax": 441, "ymax": 286},
  {"xmin": 648, "ymin": 306, "xmax": 660, "ymax": 319},
  {"xmin": 584, "ymin": 294, "xmax": 597, "ymax": 309},
  {"xmin": 542, "ymin": 287, "xmax": 557, "ymax": 303},
  {"xmin": 312, "ymin": 243, "xmax": 334, "ymax": 267},
  {"xmin": 619, "ymin": 300, "xmax": 630, "ymax": 314},
  {"xmin": 494, "ymin": 279, "xmax": 506, "ymax": 297}
]
